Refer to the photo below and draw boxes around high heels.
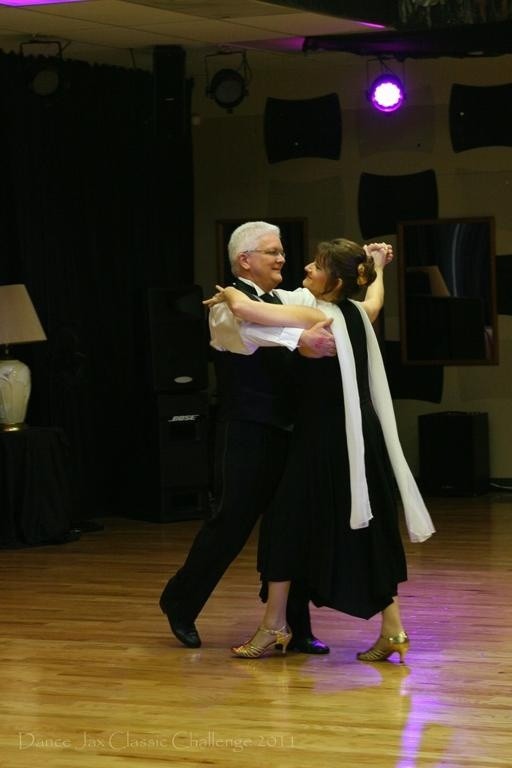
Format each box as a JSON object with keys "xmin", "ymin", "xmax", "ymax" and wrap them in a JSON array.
[
  {"xmin": 230, "ymin": 626, "xmax": 293, "ymax": 658},
  {"xmin": 357, "ymin": 630, "xmax": 410, "ymax": 664}
]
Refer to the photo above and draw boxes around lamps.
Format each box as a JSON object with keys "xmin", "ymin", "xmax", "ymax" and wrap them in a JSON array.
[
  {"xmin": 0, "ymin": 283, "xmax": 48, "ymax": 432},
  {"xmin": 366, "ymin": 55, "xmax": 407, "ymax": 113},
  {"xmin": 206, "ymin": 68, "xmax": 249, "ymax": 113}
]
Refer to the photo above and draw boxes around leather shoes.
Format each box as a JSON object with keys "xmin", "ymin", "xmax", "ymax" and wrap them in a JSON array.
[
  {"xmin": 297, "ymin": 634, "xmax": 329, "ymax": 653},
  {"xmin": 160, "ymin": 596, "xmax": 203, "ymax": 648}
]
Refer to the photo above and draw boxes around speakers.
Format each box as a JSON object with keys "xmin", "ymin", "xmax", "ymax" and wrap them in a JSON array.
[
  {"xmin": 134, "ymin": 287, "xmax": 210, "ymax": 393},
  {"xmin": 135, "ymin": 393, "xmax": 211, "ymax": 525},
  {"xmin": 418, "ymin": 411, "xmax": 491, "ymax": 498}
]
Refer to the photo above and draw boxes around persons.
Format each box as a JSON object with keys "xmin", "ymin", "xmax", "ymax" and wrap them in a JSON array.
[
  {"xmin": 153, "ymin": 219, "xmax": 396, "ymax": 650},
  {"xmin": 191, "ymin": 233, "xmax": 414, "ymax": 666},
  {"xmin": 406, "ymin": 264, "xmax": 452, "ymax": 297}
]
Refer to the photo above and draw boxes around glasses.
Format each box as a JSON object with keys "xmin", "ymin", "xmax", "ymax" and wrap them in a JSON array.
[{"xmin": 246, "ymin": 249, "xmax": 286, "ymax": 258}]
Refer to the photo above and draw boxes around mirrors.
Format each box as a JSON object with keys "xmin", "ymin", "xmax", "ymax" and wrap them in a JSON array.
[{"xmin": 396, "ymin": 214, "xmax": 500, "ymax": 366}]
